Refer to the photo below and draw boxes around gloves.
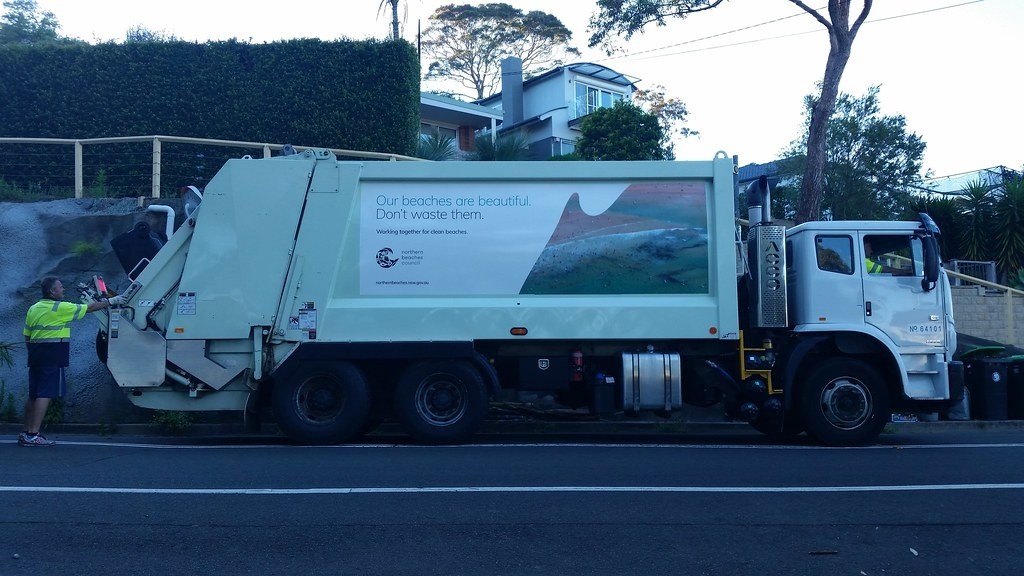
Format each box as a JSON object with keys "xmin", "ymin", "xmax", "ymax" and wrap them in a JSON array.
[{"xmin": 108, "ymin": 295, "xmax": 128, "ymax": 306}]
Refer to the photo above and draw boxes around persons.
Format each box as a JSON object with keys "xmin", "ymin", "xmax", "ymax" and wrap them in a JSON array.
[
  {"xmin": 864, "ymin": 242, "xmax": 913, "ymax": 274},
  {"xmin": 18, "ymin": 277, "xmax": 128, "ymax": 446}
]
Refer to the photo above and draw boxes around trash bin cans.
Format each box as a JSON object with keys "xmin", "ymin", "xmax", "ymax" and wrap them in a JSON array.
[
  {"xmin": 1003, "ymin": 355, "xmax": 1024, "ymax": 420},
  {"xmin": 960, "ymin": 345, "xmax": 1007, "ymax": 420},
  {"xmin": 111, "ymin": 222, "xmax": 163, "ymax": 280}
]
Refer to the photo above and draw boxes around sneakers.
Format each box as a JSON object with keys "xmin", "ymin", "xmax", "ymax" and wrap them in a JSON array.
[{"xmin": 18, "ymin": 433, "xmax": 55, "ymax": 446}]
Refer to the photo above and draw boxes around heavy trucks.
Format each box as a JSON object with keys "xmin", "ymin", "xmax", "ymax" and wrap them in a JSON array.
[{"xmin": 76, "ymin": 145, "xmax": 965, "ymax": 446}]
